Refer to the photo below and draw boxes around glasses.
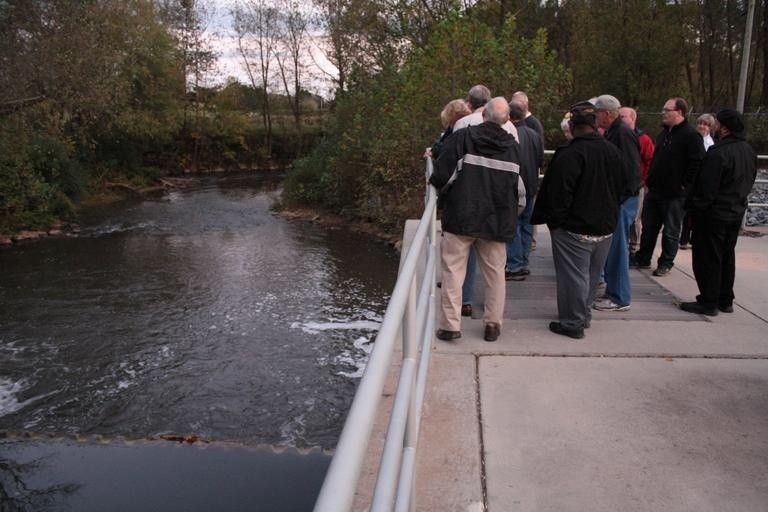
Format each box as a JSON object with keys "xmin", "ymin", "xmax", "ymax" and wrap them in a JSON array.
[{"xmin": 660, "ymin": 108, "xmax": 679, "ymax": 114}]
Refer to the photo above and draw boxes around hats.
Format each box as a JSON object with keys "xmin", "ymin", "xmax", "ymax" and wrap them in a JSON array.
[
  {"xmin": 570, "ymin": 101, "xmax": 596, "ymax": 125},
  {"xmin": 717, "ymin": 109, "xmax": 746, "ymax": 139},
  {"xmin": 594, "ymin": 95, "xmax": 621, "ymax": 112}
]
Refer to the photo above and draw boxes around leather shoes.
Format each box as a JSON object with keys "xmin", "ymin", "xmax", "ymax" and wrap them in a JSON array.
[
  {"xmin": 550, "ymin": 322, "xmax": 584, "ymax": 338},
  {"xmin": 697, "ymin": 295, "xmax": 733, "ymax": 312},
  {"xmin": 485, "ymin": 323, "xmax": 500, "ymax": 341},
  {"xmin": 437, "ymin": 329, "xmax": 461, "ymax": 340},
  {"xmin": 681, "ymin": 302, "xmax": 718, "ymax": 315},
  {"xmin": 462, "ymin": 305, "xmax": 472, "ymax": 316}
]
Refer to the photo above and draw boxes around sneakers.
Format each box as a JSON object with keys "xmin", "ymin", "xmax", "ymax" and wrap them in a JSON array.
[
  {"xmin": 680, "ymin": 243, "xmax": 692, "ymax": 249},
  {"xmin": 653, "ymin": 265, "xmax": 672, "ymax": 277},
  {"xmin": 505, "ymin": 268, "xmax": 530, "ymax": 280},
  {"xmin": 592, "ymin": 294, "xmax": 631, "ymax": 311},
  {"xmin": 629, "ymin": 260, "xmax": 651, "ymax": 269}
]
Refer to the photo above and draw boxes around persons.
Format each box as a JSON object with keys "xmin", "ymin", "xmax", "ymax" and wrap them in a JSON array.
[
  {"xmin": 679, "ymin": 109, "xmax": 759, "ymax": 316},
  {"xmin": 429, "ymin": 96, "xmax": 520, "ymax": 340},
  {"xmin": 560, "ymin": 95, "xmax": 642, "ymax": 312},
  {"xmin": 505, "ymin": 90, "xmax": 544, "ymax": 281},
  {"xmin": 626, "ymin": 97, "xmax": 705, "ymax": 275},
  {"xmin": 423, "ymin": 98, "xmax": 473, "ymax": 159},
  {"xmin": 528, "ymin": 102, "xmax": 628, "ymax": 338},
  {"xmin": 617, "ymin": 106, "xmax": 655, "ymax": 253},
  {"xmin": 679, "ymin": 114, "xmax": 716, "ymax": 251},
  {"xmin": 453, "ymin": 85, "xmax": 521, "ymax": 315}
]
